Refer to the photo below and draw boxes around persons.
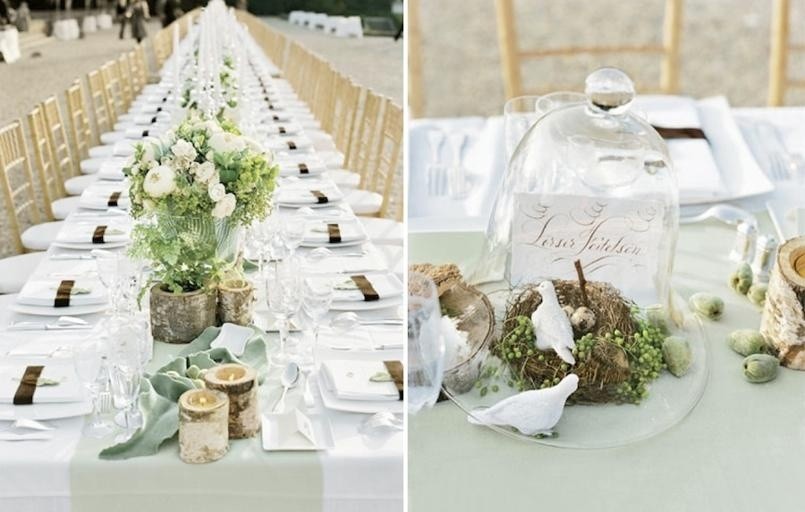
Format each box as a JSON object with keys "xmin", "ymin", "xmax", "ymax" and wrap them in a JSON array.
[
  {"xmin": 0, "ymin": 0, "xmax": 31, "ymax": 32},
  {"xmin": 112, "ymin": 0, "xmax": 183, "ymax": 44}
]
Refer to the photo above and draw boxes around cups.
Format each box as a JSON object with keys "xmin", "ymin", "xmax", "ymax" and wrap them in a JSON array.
[
  {"xmin": 499, "ymin": 89, "xmax": 588, "ymax": 158},
  {"xmin": 406, "ymin": 274, "xmax": 443, "ymax": 416}
]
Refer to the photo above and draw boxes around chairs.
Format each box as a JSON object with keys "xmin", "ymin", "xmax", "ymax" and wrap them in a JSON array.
[
  {"xmin": 408, "ymin": 0, "xmax": 805, "ymax": 119},
  {"xmin": 0, "ymin": 5, "xmax": 404, "ymax": 255}
]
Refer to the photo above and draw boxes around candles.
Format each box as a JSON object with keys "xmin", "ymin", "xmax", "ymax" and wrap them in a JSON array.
[
  {"xmin": 217, "ymin": 278, "xmax": 254, "ymax": 327},
  {"xmin": 202, "ymin": 362, "xmax": 262, "ymax": 441},
  {"xmin": 178, "ymin": 387, "xmax": 231, "ymax": 466}
]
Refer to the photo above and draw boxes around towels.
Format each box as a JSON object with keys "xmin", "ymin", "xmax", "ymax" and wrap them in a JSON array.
[
  {"xmin": 7, "ymin": 275, "xmax": 110, "ymax": 317},
  {"xmin": 319, "ymin": 269, "xmax": 404, "ymax": 311},
  {"xmin": 0, "ymin": 361, "xmax": 93, "ymax": 422},
  {"xmin": 631, "ymin": 95, "xmax": 723, "ymax": 205},
  {"xmin": 319, "ymin": 359, "xmax": 403, "ymax": 402}
]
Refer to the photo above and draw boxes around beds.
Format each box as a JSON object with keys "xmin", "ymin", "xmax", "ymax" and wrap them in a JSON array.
[
  {"xmin": 404, "ymin": 107, "xmax": 805, "ymax": 512},
  {"xmin": 0, "ymin": 2, "xmax": 403, "ymax": 512}
]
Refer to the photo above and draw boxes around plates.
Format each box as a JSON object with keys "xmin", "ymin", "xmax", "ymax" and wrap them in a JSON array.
[
  {"xmin": 0, "ymin": 180, "xmax": 139, "ymax": 425},
  {"xmin": 648, "ymin": 95, "xmax": 778, "ymax": 204},
  {"xmin": 261, "ymin": 187, "xmax": 404, "ymax": 454}
]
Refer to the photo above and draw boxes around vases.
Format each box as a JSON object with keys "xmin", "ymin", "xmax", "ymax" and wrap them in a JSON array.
[{"xmin": 146, "ymin": 275, "xmax": 218, "ymax": 344}]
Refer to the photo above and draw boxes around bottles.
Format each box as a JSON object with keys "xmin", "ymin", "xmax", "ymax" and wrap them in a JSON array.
[{"xmin": 723, "ymin": 221, "xmax": 774, "ymax": 287}]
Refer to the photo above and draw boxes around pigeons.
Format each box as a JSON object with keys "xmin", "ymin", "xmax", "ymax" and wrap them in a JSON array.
[
  {"xmin": 466, "ymin": 370, "xmax": 580, "ymax": 439},
  {"xmin": 529, "ymin": 279, "xmax": 578, "ymax": 367}
]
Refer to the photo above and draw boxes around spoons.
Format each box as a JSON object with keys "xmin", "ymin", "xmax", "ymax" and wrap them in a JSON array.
[{"xmin": 685, "ymin": 205, "xmax": 749, "ymax": 237}]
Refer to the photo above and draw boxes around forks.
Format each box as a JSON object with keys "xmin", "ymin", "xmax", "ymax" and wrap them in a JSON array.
[{"xmin": 424, "ymin": 125, "xmax": 470, "ymax": 198}]
